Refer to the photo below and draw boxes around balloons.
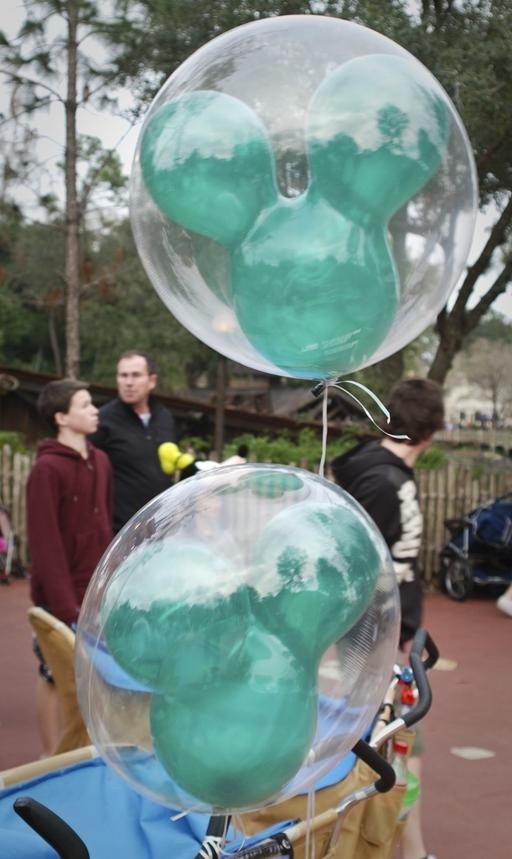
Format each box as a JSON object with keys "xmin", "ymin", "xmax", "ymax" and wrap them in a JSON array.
[
  {"xmin": 98, "ymin": 498, "xmax": 382, "ymax": 809},
  {"xmin": 65, "ymin": 459, "xmax": 407, "ymax": 817},
  {"xmin": 124, "ymin": 11, "xmax": 484, "ymax": 394},
  {"xmin": 136, "ymin": 52, "xmax": 448, "ymax": 380}
]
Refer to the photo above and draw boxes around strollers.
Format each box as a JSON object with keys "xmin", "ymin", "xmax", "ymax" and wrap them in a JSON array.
[
  {"xmin": 437, "ymin": 488, "xmax": 511, "ymax": 602},
  {"xmin": 0, "ymin": 618, "xmax": 459, "ymax": 859}
]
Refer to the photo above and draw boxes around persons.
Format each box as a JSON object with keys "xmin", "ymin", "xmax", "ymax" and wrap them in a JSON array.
[
  {"xmin": 327, "ymin": 371, "xmax": 453, "ymax": 859},
  {"xmin": 25, "ymin": 376, "xmax": 119, "ymax": 759},
  {"xmin": 82, "ymin": 345, "xmax": 188, "ymax": 541}
]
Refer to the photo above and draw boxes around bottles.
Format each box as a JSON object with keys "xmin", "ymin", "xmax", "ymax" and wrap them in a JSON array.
[{"xmin": 387, "ymin": 678, "xmax": 421, "ymax": 819}]
